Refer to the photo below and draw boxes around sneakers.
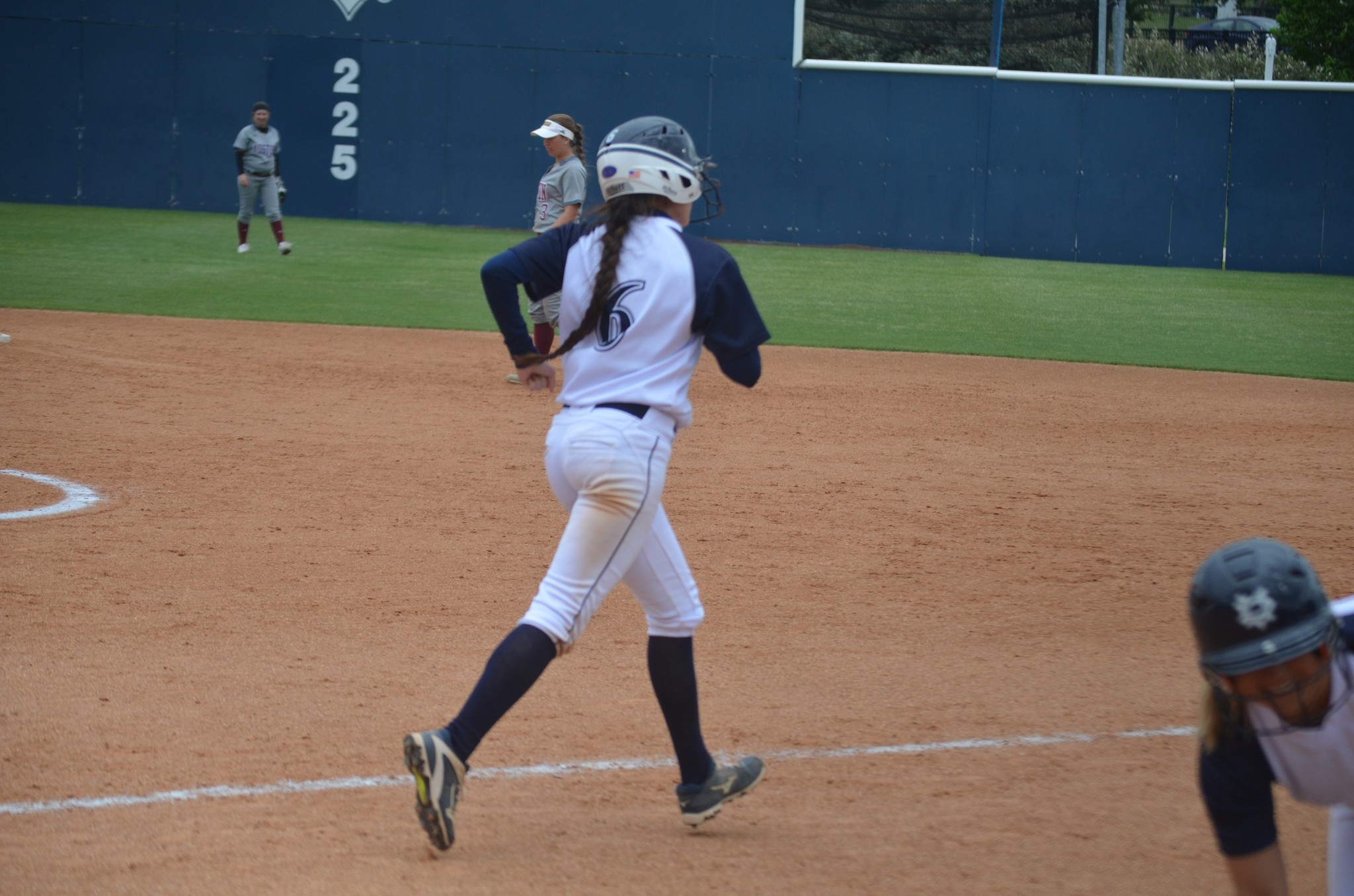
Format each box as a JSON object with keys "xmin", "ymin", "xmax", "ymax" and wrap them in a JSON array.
[
  {"xmin": 675, "ymin": 755, "xmax": 765, "ymax": 824},
  {"xmin": 401, "ymin": 731, "xmax": 469, "ymax": 851}
]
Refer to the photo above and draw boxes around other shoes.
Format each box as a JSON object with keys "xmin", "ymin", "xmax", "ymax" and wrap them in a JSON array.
[
  {"xmin": 506, "ymin": 373, "xmax": 523, "ymax": 384},
  {"xmin": 279, "ymin": 244, "xmax": 290, "ymax": 255},
  {"xmin": 237, "ymin": 243, "xmax": 248, "ymax": 253}
]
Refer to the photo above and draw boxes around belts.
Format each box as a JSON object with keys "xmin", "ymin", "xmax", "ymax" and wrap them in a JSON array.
[
  {"xmin": 562, "ymin": 402, "xmax": 676, "ymax": 433},
  {"xmin": 248, "ymin": 172, "xmax": 271, "ymax": 177}
]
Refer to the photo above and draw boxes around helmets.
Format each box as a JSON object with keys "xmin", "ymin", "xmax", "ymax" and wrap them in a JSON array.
[
  {"xmin": 596, "ymin": 116, "xmax": 703, "ymax": 204},
  {"xmin": 1185, "ymin": 537, "xmax": 1341, "ymax": 676}
]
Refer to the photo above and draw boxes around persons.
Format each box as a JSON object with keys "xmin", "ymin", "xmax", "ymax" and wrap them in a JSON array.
[
  {"xmin": 232, "ymin": 101, "xmax": 292, "ymax": 256},
  {"xmin": 507, "ymin": 114, "xmax": 587, "ymax": 385},
  {"xmin": 405, "ymin": 116, "xmax": 772, "ymax": 852}
]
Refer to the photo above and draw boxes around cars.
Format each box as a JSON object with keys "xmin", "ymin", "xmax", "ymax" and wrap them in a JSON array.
[{"xmin": 1183, "ymin": 17, "xmax": 1299, "ymax": 58}]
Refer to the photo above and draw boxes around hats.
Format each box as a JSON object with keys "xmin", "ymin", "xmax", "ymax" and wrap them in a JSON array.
[{"xmin": 530, "ymin": 119, "xmax": 576, "ymax": 144}]
